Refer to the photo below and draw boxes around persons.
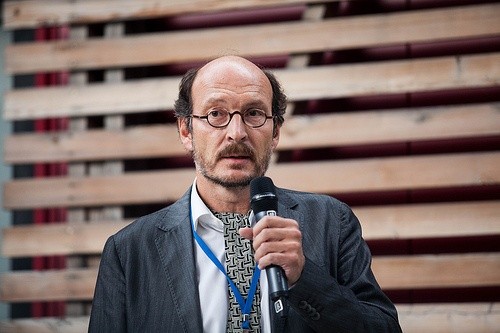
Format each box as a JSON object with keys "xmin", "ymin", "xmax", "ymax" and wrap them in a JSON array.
[{"xmin": 88, "ymin": 55, "xmax": 404, "ymax": 333}]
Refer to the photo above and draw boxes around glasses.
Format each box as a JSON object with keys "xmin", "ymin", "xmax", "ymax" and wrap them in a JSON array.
[{"xmin": 190, "ymin": 107, "xmax": 276, "ymax": 129}]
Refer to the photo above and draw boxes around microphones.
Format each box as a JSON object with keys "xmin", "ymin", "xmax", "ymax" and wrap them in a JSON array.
[{"xmin": 250, "ymin": 176, "xmax": 289, "ymax": 301}]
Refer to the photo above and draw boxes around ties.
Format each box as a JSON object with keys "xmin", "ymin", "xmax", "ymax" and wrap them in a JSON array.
[{"xmin": 214, "ymin": 211, "xmax": 265, "ymax": 333}]
